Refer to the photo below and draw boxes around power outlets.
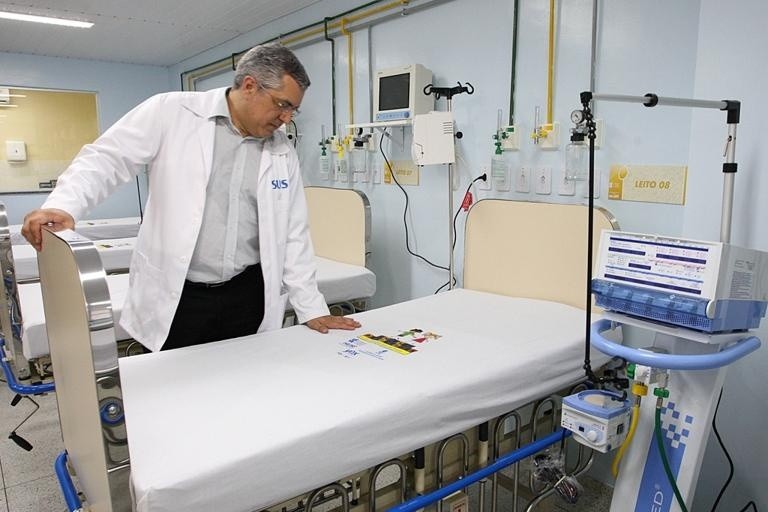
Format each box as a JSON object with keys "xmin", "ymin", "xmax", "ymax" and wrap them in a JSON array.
[
  {"xmin": 514, "ymin": 166, "xmax": 531, "ymax": 194},
  {"xmin": 535, "ymin": 164, "xmax": 553, "ymax": 195},
  {"xmin": 557, "ymin": 168, "xmax": 576, "ymax": 196},
  {"xmin": 479, "ymin": 165, "xmax": 492, "ymax": 189}
]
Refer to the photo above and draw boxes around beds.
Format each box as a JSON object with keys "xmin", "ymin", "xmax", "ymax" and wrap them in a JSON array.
[
  {"xmin": 36, "ymin": 198, "xmax": 621, "ymax": 512},
  {"xmin": 10, "ymin": 215, "xmax": 143, "ymax": 248},
  {"xmin": 0, "ymin": 185, "xmax": 376, "ymax": 452},
  {"xmin": 12, "ymin": 237, "xmax": 135, "ymax": 282}
]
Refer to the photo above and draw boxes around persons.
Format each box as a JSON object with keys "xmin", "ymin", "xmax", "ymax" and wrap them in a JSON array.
[{"xmin": 19, "ymin": 42, "xmax": 364, "ymax": 354}]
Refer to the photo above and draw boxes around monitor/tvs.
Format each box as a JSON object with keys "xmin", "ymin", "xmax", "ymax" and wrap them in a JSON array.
[{"xmin": 372, "ymin": 64, "xmax": 434, "ymax": 123}]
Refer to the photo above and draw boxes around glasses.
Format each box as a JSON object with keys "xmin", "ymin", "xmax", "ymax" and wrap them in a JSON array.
[{"xmin": 255, "ymin": 80, "xmax": 301, "ymax": 117}]
